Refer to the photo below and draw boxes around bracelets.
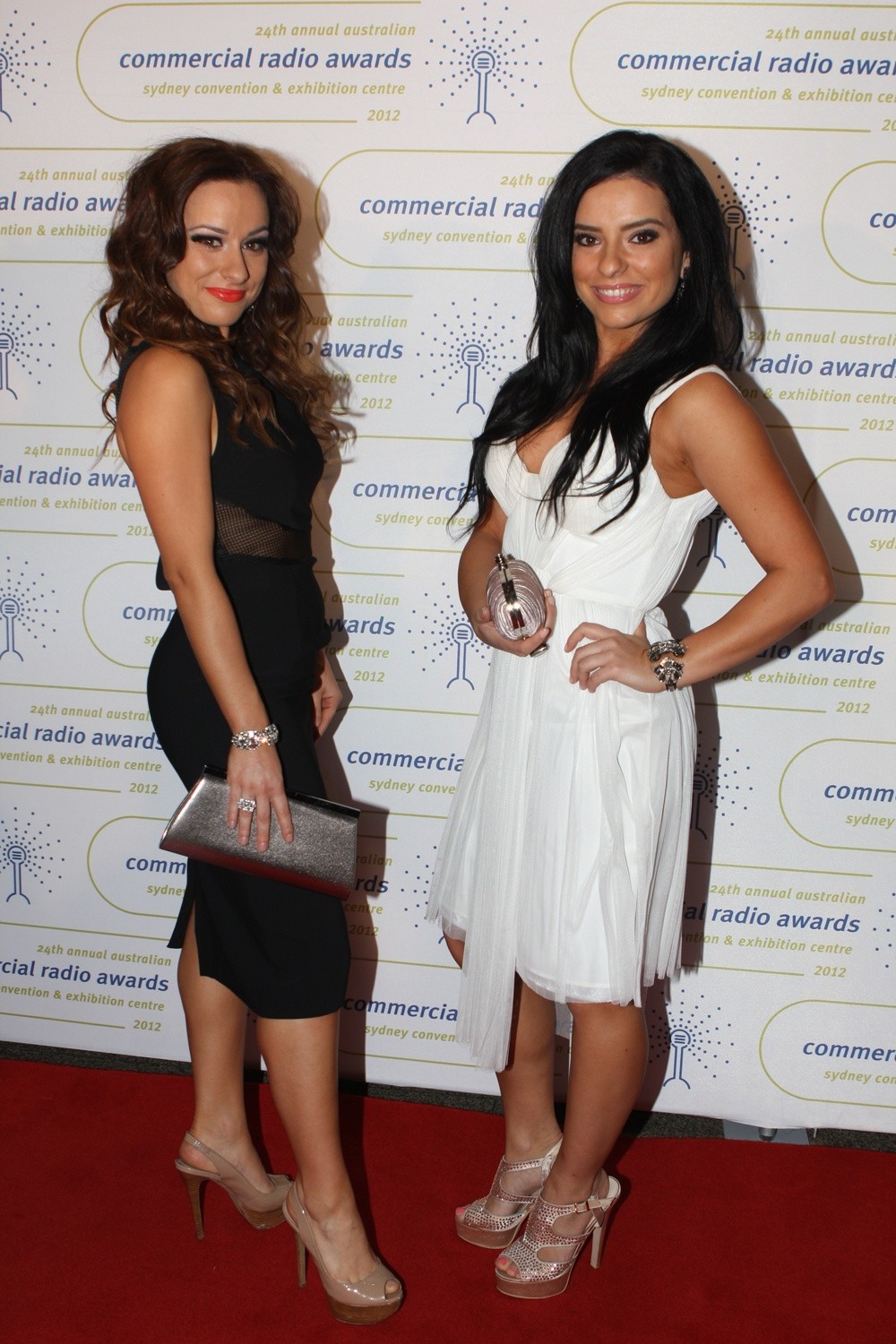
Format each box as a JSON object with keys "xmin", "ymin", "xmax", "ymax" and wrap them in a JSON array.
[
  {"xmin": 647, "ymin": 640, "xmax": 687, "ymax": 692},
  {"xmin": 231, "ymin": 724, "xmax": 278, "ymax": 750}
]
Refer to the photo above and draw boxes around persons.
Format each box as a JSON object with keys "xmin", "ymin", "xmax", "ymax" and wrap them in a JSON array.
[
  {"xmin": 88, "ymin": 137, "xmax": 403, "ymax": 1325},
  {"xmin": 423, "ymin": 128, "xmax": 839, "ymax": 1298}
]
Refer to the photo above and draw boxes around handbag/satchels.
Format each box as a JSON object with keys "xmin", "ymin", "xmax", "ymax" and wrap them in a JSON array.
[{"xmin": 159, "ymin": 767, "xmax": 359, "ymax": 899}]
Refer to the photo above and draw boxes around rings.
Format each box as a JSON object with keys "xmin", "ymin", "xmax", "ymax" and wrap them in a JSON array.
[
  {"xmin": 238, "ymin": 797, "xmax": 256, "ymax": 812},
  {"xmin": 529, "ymin": 645, "xmax": 548, "ymax": 657}
]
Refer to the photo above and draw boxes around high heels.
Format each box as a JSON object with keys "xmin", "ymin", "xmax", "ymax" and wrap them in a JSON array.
[
  {"xmin": 174, "ymin": 1131, "xmax": 296, "ymax": 1240},
  {"xmin": 281, "ymin": 1176, "xmax": 403, "ymax": 1323},
  {"xmin": 454, "ymin": 1135, "xmax": 565, "ymax": 1249},
  {"xmin": 494, "ymin": 1172, "xmax": 622, "ymax": 1300}
]
